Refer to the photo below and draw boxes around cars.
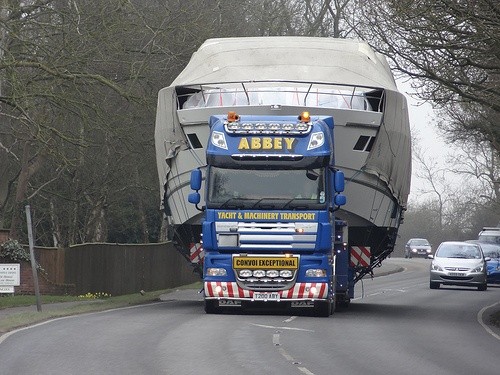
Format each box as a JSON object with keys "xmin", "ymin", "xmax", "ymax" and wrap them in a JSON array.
[
  {"xmin": 405, "ymin": 238, "xmax": 433, "ymax": 259},
  {"xmin": 466, "ymin": 239, "xmax": 500, "ymax": 285},
  {"xmin": 430, "ymin": 241, "xmax": 491, "ymax": 290}
]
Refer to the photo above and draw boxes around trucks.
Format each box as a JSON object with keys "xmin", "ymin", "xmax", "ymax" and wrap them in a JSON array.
[
  {"xmin": 190, "ymin": 112, "xmax": 371, "ymax": 316},
  {"xmin": 479, "ymin": 227, "xmax": 500, "ymax": 244}
]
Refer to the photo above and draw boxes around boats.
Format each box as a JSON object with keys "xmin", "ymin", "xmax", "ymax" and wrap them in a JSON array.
[{"xmin": 153, "ymin": 37, "xmax": 412, "ymax": 246}]
